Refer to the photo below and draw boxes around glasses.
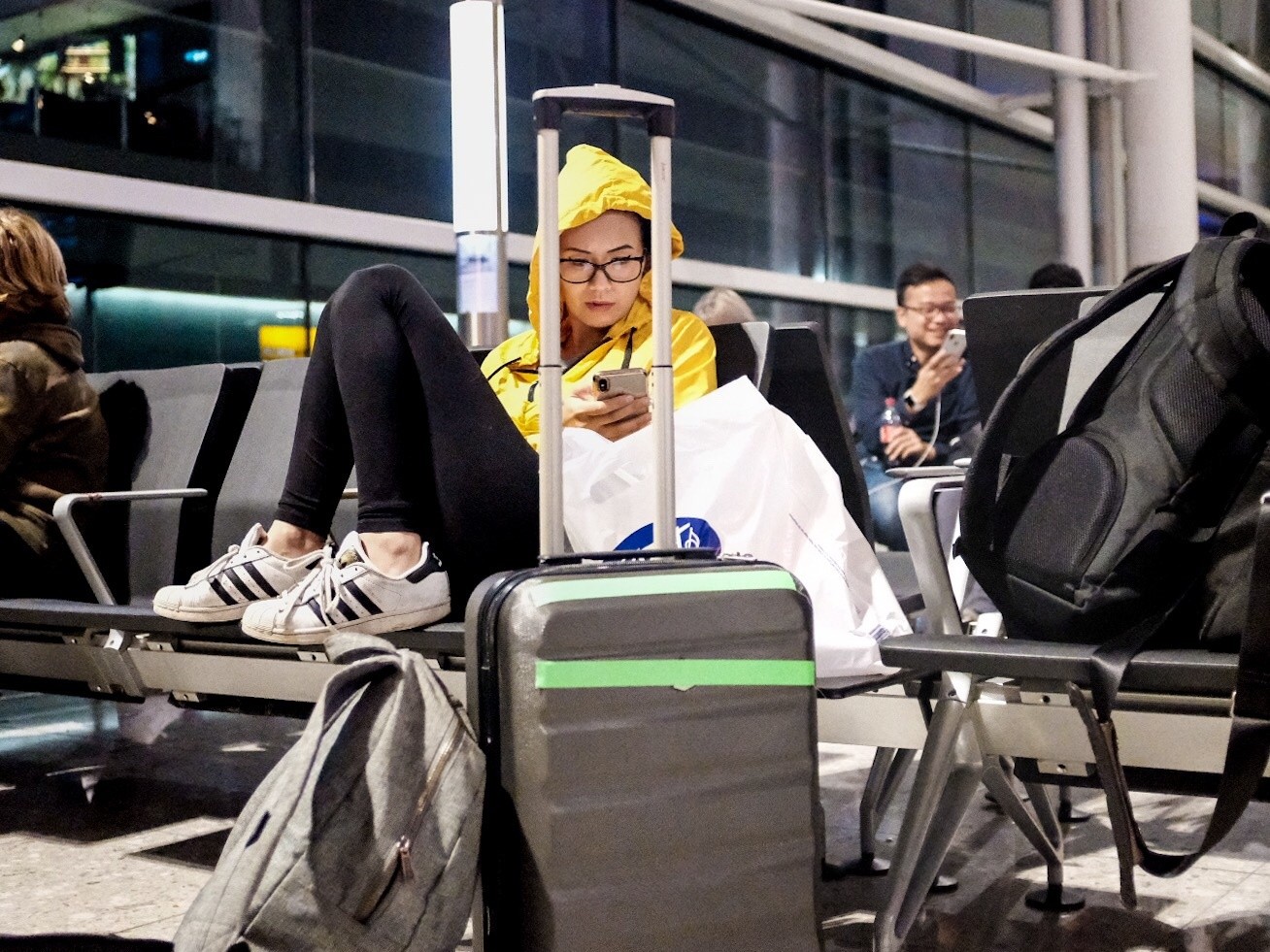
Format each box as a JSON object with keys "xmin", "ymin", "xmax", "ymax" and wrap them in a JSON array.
[
  {"xmin": 557, "ymin": 247, "xmax": 647, "ymax": 284},
  {"xmin": 900, "ymin": 303, "xmax": 962, "ymax": 320}
]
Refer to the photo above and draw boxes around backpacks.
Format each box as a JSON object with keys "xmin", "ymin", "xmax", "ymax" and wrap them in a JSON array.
[
  {"xmin": 951, "ymin": 210, "xmax": 1270, "ymax": 652},
  {"xmin": 173, "ymin": 632, "xmax": 485, "ymax": 952}
]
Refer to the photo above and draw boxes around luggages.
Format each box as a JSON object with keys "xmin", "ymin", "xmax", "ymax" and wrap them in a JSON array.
[{"xmin": 461, "ymin": 81, "xmax": 826, "ymax": 952}]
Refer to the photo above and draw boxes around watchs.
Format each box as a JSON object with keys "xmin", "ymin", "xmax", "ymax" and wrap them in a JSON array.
[{"xmin": 902, "ymin": 388, "xmax": 926, "ymax": 413}]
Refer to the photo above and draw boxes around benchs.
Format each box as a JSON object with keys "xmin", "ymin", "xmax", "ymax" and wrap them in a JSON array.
[{"xmin": 1, "ymin": 319, "xmax": 1270, "ymax": 952}]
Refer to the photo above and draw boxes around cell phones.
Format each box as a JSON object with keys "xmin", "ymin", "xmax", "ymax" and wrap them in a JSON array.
[
  {"xmin": 592, "ymin": 367, "xmax": 648, "ymax": 422},
  {"xmin": 941, "ymin": 328, "xmax": 966, "ymax": 358}
]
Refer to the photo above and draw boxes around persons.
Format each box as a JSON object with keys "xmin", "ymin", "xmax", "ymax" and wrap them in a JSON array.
[
  {"xmin": 691, "ymin": 286, "xmax": 756, "ymax": 328},
  {"xmin": 153, "ymin": 144, "xmax": 717, "ymax": 652},
  {"xmin": 1028, "ymin": 263, "xmax": 1085, "ymax": 288},
  {"xmin": 0, "ymin": 205, "xmax": 109, "ymax": 597},
  {"xmin": 850, "ymin": 263, "xmax": 981, "ymax": 552}
]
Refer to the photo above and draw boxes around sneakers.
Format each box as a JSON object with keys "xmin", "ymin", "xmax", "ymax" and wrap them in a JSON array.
[
  {"xmin": 150, "ymin": 520, "xmax": 340, "ymax": 623},
  {"xmin": 238, "ymin": 528, "xmax": 454, "ymax": 645}
]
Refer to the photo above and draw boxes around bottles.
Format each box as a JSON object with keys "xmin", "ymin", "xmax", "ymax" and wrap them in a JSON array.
[{"xmin": 880, "ymin": 397, "xmax": 902, "ymax": 467}]
